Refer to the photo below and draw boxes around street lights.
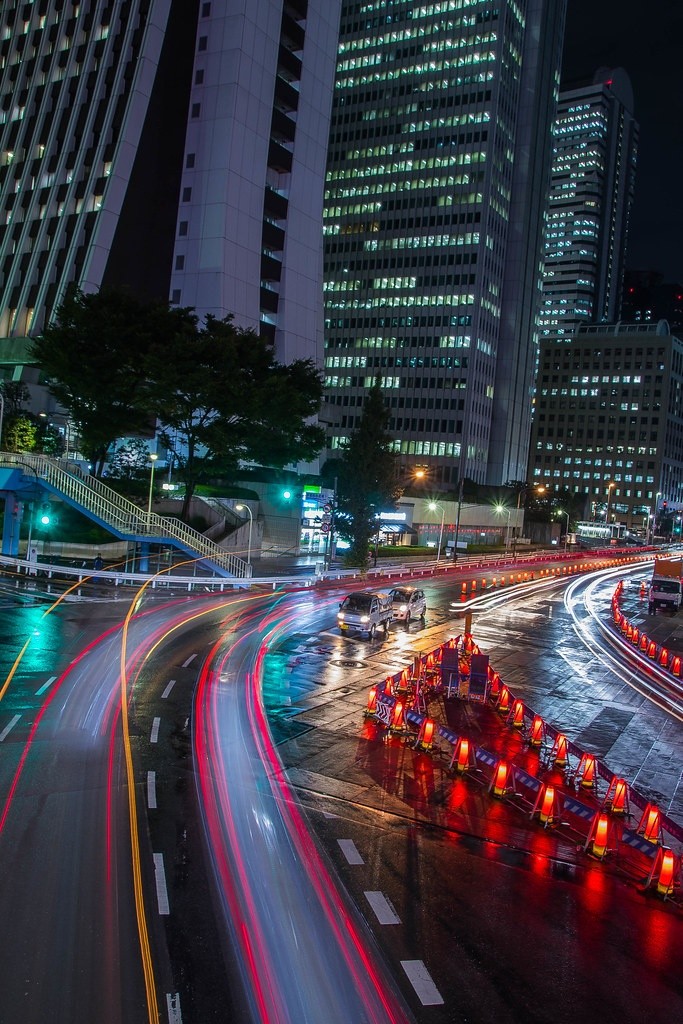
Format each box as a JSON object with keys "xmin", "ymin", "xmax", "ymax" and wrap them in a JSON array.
[
  {"xmin": 557, "ymin": 509, "xmax": 569, "ymax": 553},
  {"xmin": 145, "ymin": 452, "xmax": 158, "ymax": 533},
  {"xmin": 513, "ymin": 484, "xmax": 546, "ymax": 564},
  {"xmin": 235, "ymin": 503, "xmax": 253, "ymax": 565},
  {"xmin": 607, "ymin": 482, "xmax": 616, "ymax": 524},
  {"xmin": 494, "ymin": 503, "xmax": 511, "ymax": 553},
  {"xmin": 428, "ymin": 502, "xmax": 447, "ymax": 562}
]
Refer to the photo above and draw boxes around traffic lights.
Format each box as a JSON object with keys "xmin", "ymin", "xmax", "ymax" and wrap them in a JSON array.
[
  {"xmin": 40, "ymin": 501, "xmax": 52, "ymax": 527},
  {"xmin": 282, "ymin": 490, "xmax": 292, "ymax": 505}
]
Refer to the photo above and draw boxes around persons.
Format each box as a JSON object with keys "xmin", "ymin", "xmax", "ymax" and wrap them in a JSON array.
[
  {"xmin": 361, "ymin": 601, "xmax": 375, "ymax": 613},
  {"xmin": 398, "ymin": 595, "xmax": 405, "ymax": 600},
  {"xmin": 648, "ymin": 588, "xmax": 657, "ymax": 615},
  {"xmin": 92, "ymin": 553, "xmax": 103, "ymax": 584},
  {"xmin": 350, "ymin": 599, "xmax": 358, "ymax": 609}
]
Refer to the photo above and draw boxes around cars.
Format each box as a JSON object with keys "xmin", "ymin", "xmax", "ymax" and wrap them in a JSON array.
[{"xmin": 388, "ymin": 585, "xmax": 427, "ymax": 624}]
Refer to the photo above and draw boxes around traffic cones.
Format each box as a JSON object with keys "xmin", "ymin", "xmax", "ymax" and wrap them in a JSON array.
[
  {"xmin": 657, "ymin": 849, "xmax": 677, "ymax": 899},
  {"xmin": 610, "ymin": 577, "xmax": 683, "ymax": 679},
  {"xmin": 610, "ymin": 778, "xmax": 625, "ymax": 816},
  {"xmin": 591, "ymin": 812, "xmax": 608, "ymax": 859},
  {"xmin": 362, "ymin": 631, "xmax": 570, "ymax": 801},
  {"xmin": 536, "ymin": 785, "xmax": 555, "ymax": 826},
  {"xmin": 644, "ymin": 805, "xmax": 662, "ymax": 846},
  {"xmin": 460, "ymin": 551, "xmax": 682, "ymax": 594},
  {"xmin": 578, "ymin": 755, "xmax": 595, "ymax": 789}
]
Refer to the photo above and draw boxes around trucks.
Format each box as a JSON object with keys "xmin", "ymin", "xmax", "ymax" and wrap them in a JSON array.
[
  {"xmin": 649, "ymin": 573, "xmax": 683, "ymax": 611},
  {"xmin": 337, "ymin": 590, "xmax": 394, "ymax": 636}
]
